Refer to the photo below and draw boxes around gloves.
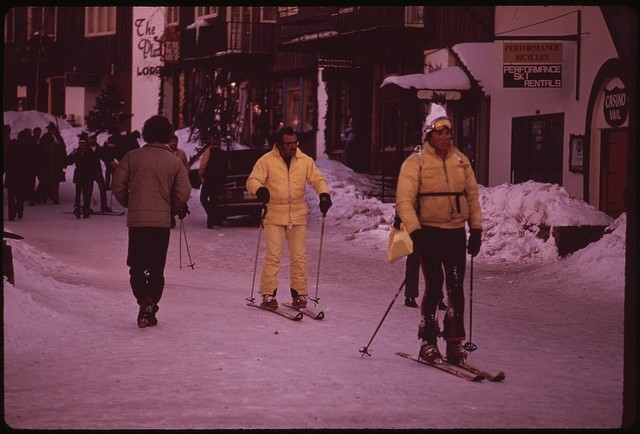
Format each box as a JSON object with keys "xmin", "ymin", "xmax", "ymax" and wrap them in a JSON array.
[
  {"xmin": 320, "ymin": 193, "xmax": 332, "ymax": 213},
  {"xmin": 256, "ymin": 187, "xmax": 269, "ymax": 203},
  {"xmin": 393, "ymin": 217, "xmax": 402, "ymax": 230}
]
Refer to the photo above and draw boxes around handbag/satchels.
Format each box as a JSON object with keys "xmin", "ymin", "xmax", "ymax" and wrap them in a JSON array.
[{"xmin": 387, "ymin": 222, "xmax": 413, "ymax": 264}]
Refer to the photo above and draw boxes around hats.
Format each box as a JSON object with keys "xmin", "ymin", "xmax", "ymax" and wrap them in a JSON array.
[
  {"xmin": 46, "ymin": 122, "xmax": 58, "ymax": 130},
  {"xmin": 422, "ymin": 103, "xmax": 447, "ymax": 143}
]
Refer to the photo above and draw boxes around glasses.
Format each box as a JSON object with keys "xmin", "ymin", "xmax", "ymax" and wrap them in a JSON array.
[
  {"xmin": 424, "ymin": 118, "xmax": 452, "ymax": 132},
  {"xmin": 284, "ymin": 141, "xmax": 299, "ymax": 147}
]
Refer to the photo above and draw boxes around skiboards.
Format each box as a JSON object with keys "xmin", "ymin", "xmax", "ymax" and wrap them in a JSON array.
[
  {"xmin": 247, "ymin": 302, "xmax": 324, "ymax": 321},
  {"xmin": 62, "ymin": 210, "xmax": 125, "ymax": 216},
  {"xmin": 396, "ymin": 351, "xmax": 506, "ymax": 381}
]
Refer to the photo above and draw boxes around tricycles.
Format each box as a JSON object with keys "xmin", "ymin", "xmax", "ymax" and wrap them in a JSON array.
[{"xmin": 210, "ymin": 149, "xmax": 271, "ymax": 224}]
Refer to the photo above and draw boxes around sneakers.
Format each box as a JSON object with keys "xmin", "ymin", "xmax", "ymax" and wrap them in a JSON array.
[
  {"xmin": 446, "ymin": 344, "xmax": 467, "ymax": 364},
  {"xmin": 74, "ymin": 209, "xmax": 80, "ymax": 219},
  {"xmin": 438, "ymin": 302, "xmax": 447, "ymax": 310},
  {"xmin": 137, "ymin": 298, "xmax": 159, "ymax": 328},
  {"xmin": 101, "ymin": 206, "xmax": 112, "ymax": 212},
  {"xmin": 420, "ymin": 344, "xmax": 443, "ymax": 365},
  {"xmin": 468, "ymin": 229, "xmax": 483, "ymax": 256},
  {"xmin": 293, "ymin": 296, "xmax": 307, "ymax": 309},
  {"xmin": 83, "ymin": 209, "xmax": 90, "ymax": 218},
  {"xmin": 207, "ymin": 224, "xmax": 213, "ymax": 228},
  {"xmin": 263, "ymin": 294, "xmax": 278, "ymax": 309},
  {"xmin": 405, "ymin": 298, "xmax": 418, "ymax": 308}
]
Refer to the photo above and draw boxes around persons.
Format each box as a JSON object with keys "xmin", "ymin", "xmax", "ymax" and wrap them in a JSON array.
[
  {"xmin": 393, "ymin": 212, "xmax": 450, "ymax": 310},
  {"xmin": 113, "ymin": 130, "xmax": 140, "ymax": 163},
  {"xmin": 245, "ymin": 126, "xmax": 333, "ymax": 308},
  {"xmin": 167, "ymin": 135, "xmax": 191, "ymax": 228},
  {"xmin": 101, "ymin": 128, "xmax": 121, "ymax": 190},
  {"xmin": 82, "ymin": 130, "xmax": 119, "ymax": 215},
  {"xmin": 395, "ymin": 102, "xmax": 484, "ymax": 365},
  {"xmin": 63, "ymin": 136, "xmax": 101, "ymax": 218},
  {"xmin": 112, "ymin": 115, "xmax": 191, "ymax": 328},
  {"xmin": 198, "ymin": 135, "xmax": 232, "ymax": 229},
  {"xmin": 1, "ymin": 122, "xmax": 67, "ymax": 221}
]
